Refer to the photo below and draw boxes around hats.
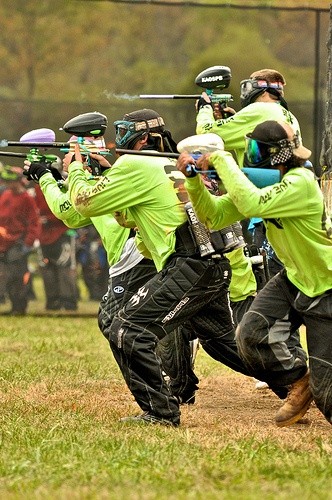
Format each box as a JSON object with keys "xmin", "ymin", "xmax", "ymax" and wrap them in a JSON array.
[{"xmin": 276, "ymin": 121, "xmax": 312, "ymax": 159}]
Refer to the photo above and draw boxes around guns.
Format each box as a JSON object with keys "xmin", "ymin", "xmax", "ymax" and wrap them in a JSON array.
[
  {"xmin": 139, "ymin": 65, "xmax": 235, "ymax": 120},
  {"xmin": 114, "ymin": 132, "xmax": 281, "ymax": 189},
  {"xmin": 7, "ymin": 111, "xmax": 113, "ymax": 177},
  {"xmin": 0, "ymin": 128, "xmax": 66, "ymax": 190}
]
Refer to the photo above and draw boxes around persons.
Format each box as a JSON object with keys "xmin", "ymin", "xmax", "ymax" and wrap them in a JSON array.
[
  {"xmin": 0, "ymin": 168, "xmax": 108, "ymax": 316},
  {"xmin": 23, "ymin": 69, "xmax": 332, "ymax": 425}
]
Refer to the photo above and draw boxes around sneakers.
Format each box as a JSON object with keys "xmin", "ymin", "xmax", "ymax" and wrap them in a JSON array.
[
  {"xmin": 274, "ymin": 368, "xmax": 314, "ymax": 428},
  {"xmin": 173, "ymin": 390, "xmax": 196, "ymax": 404},
  {"xmin": 120, "ymin": 410, "xmax": 180, "ymax": 428}
]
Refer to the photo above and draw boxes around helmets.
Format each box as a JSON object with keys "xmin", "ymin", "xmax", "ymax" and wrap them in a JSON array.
[
  {"xmin": 242, "ymin": 121, "xmax": 293, "ymax": 169},
  {"xmin": 113, "ymin": 107, "xmax": 165, "ymax": 156},
  {"xmin": 45, "ymin": 155, "xmax": 62, "ymax": 172},
  {"xmin": 0, "ymin": 165, "xmax": 31, "ymax": 188},
  {"xmin": 68, "ymin": 134, "xmax": 107, "ymax": 162}
]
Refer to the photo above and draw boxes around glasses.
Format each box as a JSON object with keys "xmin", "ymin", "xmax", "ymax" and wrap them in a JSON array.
[
  {"xmin": 239, "ymin": 79, "xmax": 257, "ymax": 99},
  {"xmin": 114, "ymin": 121, "xmax": 135, "ymax": 145}
]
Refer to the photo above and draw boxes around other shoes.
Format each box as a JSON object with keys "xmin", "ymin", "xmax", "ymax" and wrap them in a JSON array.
[
  {"xmin": 44, "ymin": 297, "xmax": 78, "ymax": 310},
  {"xmin": 7, "ymin": 296, "xmax": 28, "ymax": 316}
]
